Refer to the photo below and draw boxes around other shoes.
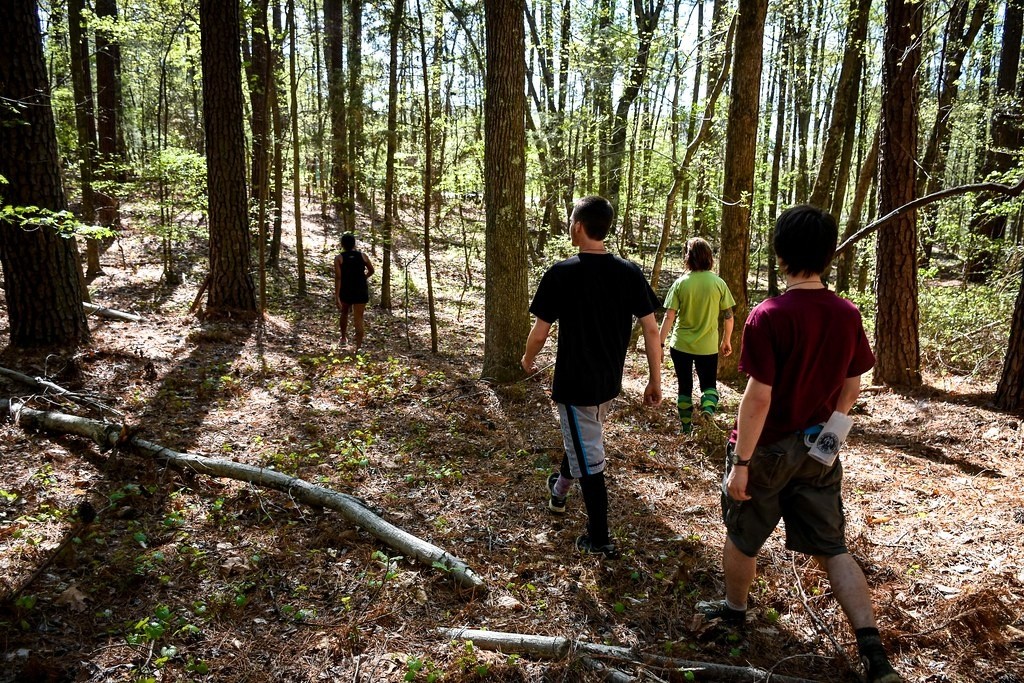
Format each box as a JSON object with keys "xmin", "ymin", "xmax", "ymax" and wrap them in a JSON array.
[
  {"xmin": 701, "ymin": 410, "xmax": 722, "ymax": 443},
  {"xmin": 684, "ymin": 425, "xmax": 692, "ymax": 434}
]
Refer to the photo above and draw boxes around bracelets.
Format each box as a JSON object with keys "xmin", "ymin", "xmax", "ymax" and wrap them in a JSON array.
[{"xmin": 661, "ymin": 343, "xmax": 665, "ymax": 348}]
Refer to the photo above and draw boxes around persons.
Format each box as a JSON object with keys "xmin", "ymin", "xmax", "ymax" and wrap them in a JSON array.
[
  {"xmin": 689, "ymin": 205, "xmax": 913, "ymax": 683},
  {"xmin": 520, "ymin": 196, "xmax": 664, "ymax": 560},
  {"xmin": 653, "ymin": 236, "xmax": 735, "ymax": 434},
  {"xmin": 334, "ymin": 232, "xmax": 375, "ymax": 355}
]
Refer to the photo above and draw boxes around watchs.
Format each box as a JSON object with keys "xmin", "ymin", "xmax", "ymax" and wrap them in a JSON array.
[{"xmin": 731, "ymin": 454, "xmax": 750, "ymax": 466}]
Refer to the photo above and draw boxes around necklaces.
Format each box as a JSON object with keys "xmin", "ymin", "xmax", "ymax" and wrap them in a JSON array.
[{"xmin": 580, "ymin": 248, "xmax": 606, "ymax": 254}]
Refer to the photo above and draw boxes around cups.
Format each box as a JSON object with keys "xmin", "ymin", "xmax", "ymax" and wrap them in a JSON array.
[{"xmin": 804, "ymin": 411, "xmax": 853, "ymax": 466}]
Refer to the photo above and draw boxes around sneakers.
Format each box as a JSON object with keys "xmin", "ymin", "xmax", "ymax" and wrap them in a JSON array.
[
  {"xmin": 547, "ymin": 473, "xmax": 566, "ymax": 512},
  {"xmin": 861, "ymin": 663, "xmax": 900, "ymax": 683},
  {"xmin": 694, "ymin": 600, "xmax": 747, "ymax": 623},
  {"xmin": 338, "ymin": 336, "xmax": 348, "ymax": 346},
  {"xmin": 575, "ymin": 534, "xmax": 618, "ymax": 559}
]
